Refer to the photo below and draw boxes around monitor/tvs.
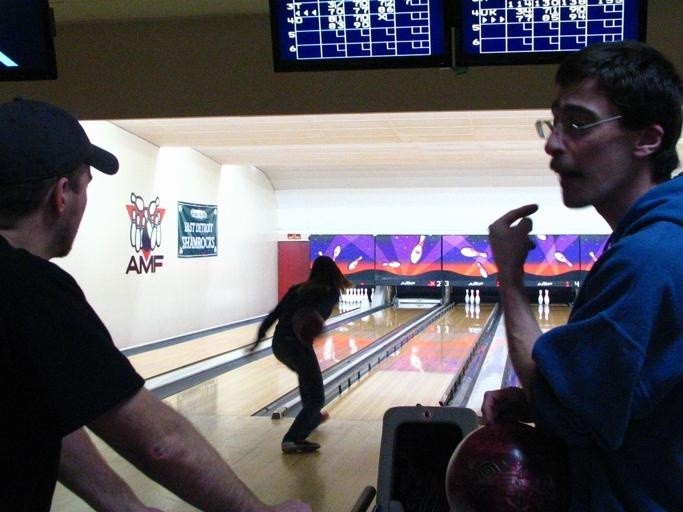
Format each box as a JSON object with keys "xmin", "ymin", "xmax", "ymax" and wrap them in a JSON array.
[
  {"xmin": 457, "ymin": 0, "xmax": 647, "ymax": 66},
  {"xmin": 270, "ymin": 0, "xmax": 454, "ymax": 74},
  {"xmin": 0, "ymin": 0, "xmax": 63, "ymax": 83}
]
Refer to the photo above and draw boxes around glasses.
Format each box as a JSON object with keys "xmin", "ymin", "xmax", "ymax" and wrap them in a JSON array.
[{"xmin": 535, "ymin": 113, "xmax": 622, "ymax": 139}]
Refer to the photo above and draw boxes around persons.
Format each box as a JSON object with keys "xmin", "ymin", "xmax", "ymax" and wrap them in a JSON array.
[
  {"xmin": 486, "ymin": 38, "xmax": 682, "ymax": 511},
  {"xmin": 247, "ymin": 256, "xmax": 354, "ymax": 455},
  {"xmin": 1, "ymin": 95, "xmax": 312, "ymax": 512}
]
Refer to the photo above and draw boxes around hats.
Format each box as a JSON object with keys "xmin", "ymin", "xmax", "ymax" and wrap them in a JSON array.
[{"xmin": 0, "ymin": 97, "xmax": 118, "ymax": 184}]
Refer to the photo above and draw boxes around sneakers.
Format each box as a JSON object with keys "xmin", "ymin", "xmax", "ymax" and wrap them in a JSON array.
[{"xmin": 282, "ymin": 439, "xmax": 321, "ymax": 453}]
